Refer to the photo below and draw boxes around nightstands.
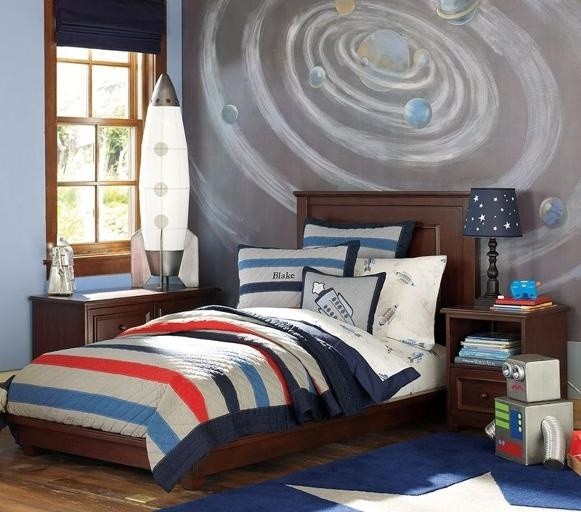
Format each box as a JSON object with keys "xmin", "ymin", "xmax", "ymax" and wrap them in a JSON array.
[{"xmin": 440, "ymin": 308, "xmax": 568, "ymax": 433}]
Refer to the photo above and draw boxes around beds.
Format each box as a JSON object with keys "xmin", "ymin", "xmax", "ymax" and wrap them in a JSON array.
[{"xmin": 6, "ymin": 190, "xmax": 480, "ymax": 492}]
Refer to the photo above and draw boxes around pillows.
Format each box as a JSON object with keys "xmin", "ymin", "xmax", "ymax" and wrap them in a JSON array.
[{"xmin": 232, "ymin": 216, "xmax": 448, "ymax": 351}]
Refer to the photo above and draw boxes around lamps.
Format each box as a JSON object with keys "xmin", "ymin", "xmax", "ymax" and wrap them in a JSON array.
[
  {"xmin": 130, "ymin": 69, "xmax": 200, "ymax": 292},
  {"xmin": 461, "ymin": 188, "xmax": 523, "ymax": 306}
]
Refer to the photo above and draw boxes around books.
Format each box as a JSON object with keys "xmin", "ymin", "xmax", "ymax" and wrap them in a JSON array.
[
  {"xmin": 454, "ymin": 333, "xmax": 521, "ymax": 367},
  {"xmin": 489, "ymin": 295, "xmax": 557, "ymax": 313}
]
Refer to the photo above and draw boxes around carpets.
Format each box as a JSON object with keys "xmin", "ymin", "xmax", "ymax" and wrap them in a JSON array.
[{"xmin": 150, "ymin": 431, "xmax": 581, "ymax": 512}]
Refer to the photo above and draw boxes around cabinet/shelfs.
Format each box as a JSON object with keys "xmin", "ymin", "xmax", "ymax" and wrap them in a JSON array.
[{"xmin": 29, "ymin": 290, "xmax": 220, "ymax": 360}]
